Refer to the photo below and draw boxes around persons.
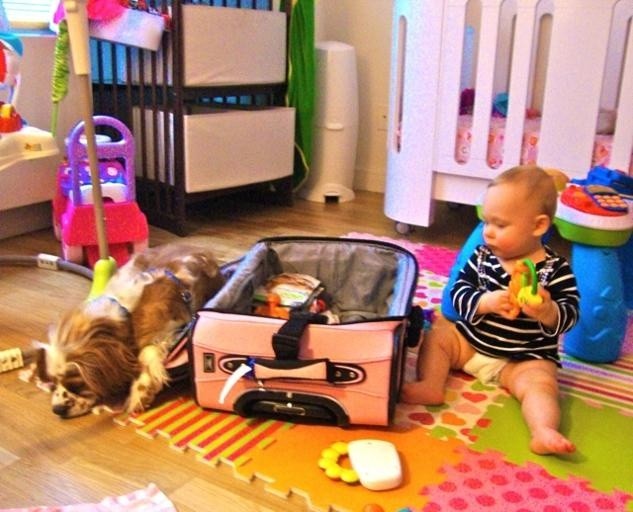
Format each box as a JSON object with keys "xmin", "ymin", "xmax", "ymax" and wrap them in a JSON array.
[{"xmin": 398, "ymin": 167, "xmax": 580, "ymax": 456}]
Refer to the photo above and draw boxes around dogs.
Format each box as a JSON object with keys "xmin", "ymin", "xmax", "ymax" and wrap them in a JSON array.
[{"xmin": 32, "ymin": 244, "xmax": 226, "ymax": 420}]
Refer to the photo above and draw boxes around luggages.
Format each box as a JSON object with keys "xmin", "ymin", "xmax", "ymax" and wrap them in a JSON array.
[{"xmin": 167, "ymin": 235, "xmax": 424, "ymax": 428}]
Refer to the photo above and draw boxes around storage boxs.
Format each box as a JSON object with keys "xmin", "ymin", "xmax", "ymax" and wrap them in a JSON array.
[
  {"xmin": 90, "ymin": 5, "xmax": 287, "ymax": 86},
  {"xmin": 133, "ymin": 105, "xmax": 298, "ymax": 190}
]
